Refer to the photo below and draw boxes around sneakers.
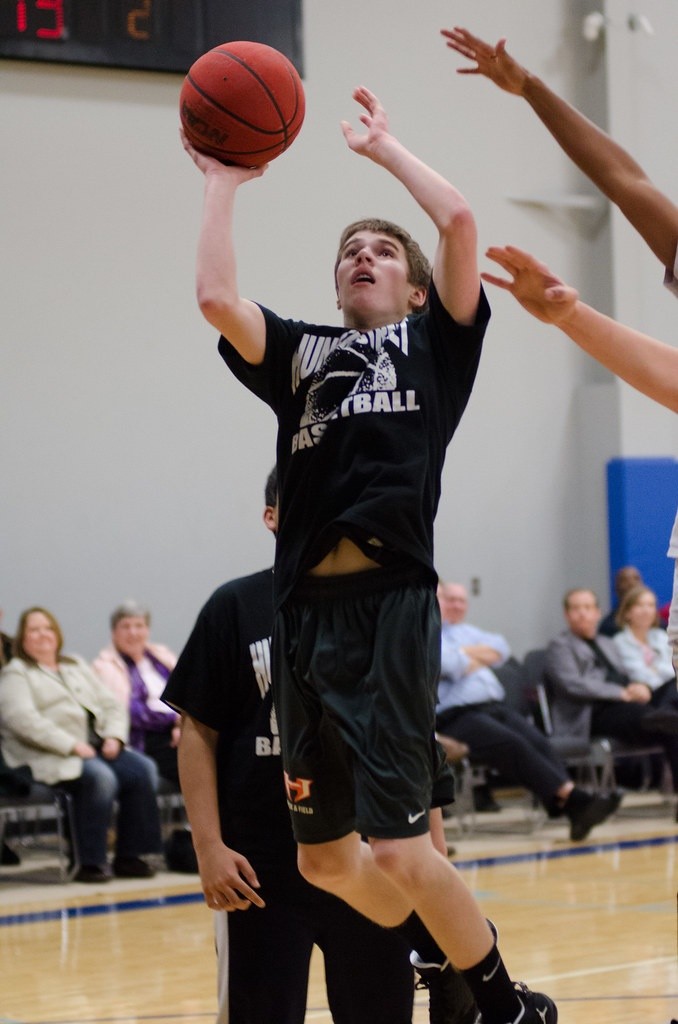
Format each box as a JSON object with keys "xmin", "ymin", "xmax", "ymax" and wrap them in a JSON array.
[
  {"xmin": 410, "ymin": 950, "xmax": 481, "ymax": 1024},
  {"xmin": 481, "ymin": 981, "xmax": 558, "ymax": 1024}
]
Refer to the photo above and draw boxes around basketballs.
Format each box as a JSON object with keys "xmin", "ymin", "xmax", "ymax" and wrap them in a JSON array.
[{"xmin": 179, "ymin": 40, "xmax": 306, "ymax": 167}]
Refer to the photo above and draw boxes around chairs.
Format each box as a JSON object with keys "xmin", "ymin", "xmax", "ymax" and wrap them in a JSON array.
[{"xmin": 1, "ymin": 651, "xmax": 670, "ymax": 886}]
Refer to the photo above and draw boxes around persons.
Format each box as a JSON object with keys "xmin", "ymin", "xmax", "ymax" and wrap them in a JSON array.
[
  {"xmin": 432, "ymin": 566, "xmax": 678, "ymax": 858},
  {"xmin": 180, "ymin": 86, "xmax": 557, "ymax": 1024},
  {"xmin": 441, "ymin": 26, "xmax": 678, "ymax": 676},
  {"xmin": 0, "ymin": 601, "xmax": 199, "ymax": 881},
  {"xmin": 162, "ymin": 462, "xmax": 456, "ymax": 1024}
]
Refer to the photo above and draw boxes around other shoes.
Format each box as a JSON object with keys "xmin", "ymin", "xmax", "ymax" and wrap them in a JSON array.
[
  {"xmin": 471, "ymin": 784, "xmax": 501, "ymax": 813},
  {"xmin": 163, "ymin": 830, "xmax": 200, "ymax": 873},
  {"xmin": 571, "ymin": 788, "xmax": 624, "ymax": 840},
  {"xmin": 111, "ymin": 857, "xmax": 154, "ymax": 879},
  {"xmin": 67, "ymin": 863, "xmax": 109, "ymax": 883}
]
